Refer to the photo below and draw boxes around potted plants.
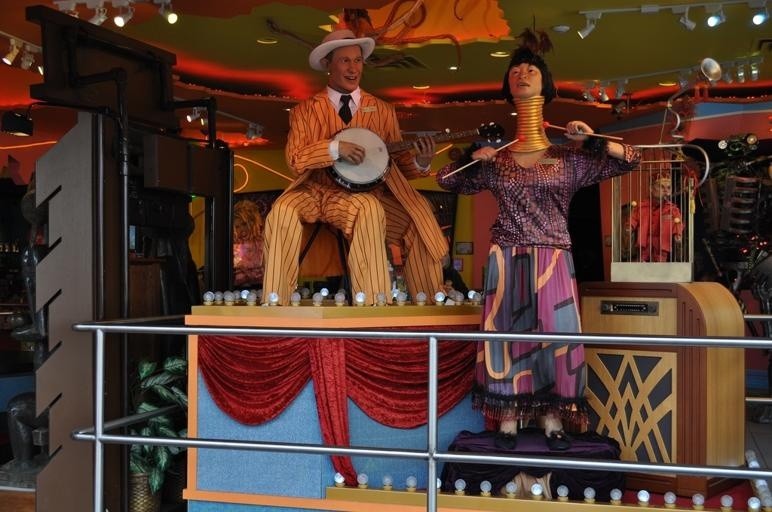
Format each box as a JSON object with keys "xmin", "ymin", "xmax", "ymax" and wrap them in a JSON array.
[{"xmin": 128, "ymin": 357, "xmax": 187, "ymax": 512}]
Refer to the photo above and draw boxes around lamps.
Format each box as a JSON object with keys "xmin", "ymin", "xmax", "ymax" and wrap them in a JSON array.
[
  {"xmin": 577, "ymin": 0, "xmax": 770, "ymax": 112},
  {"xmin": 0, "ymin": 0, "xmax": 265, "ymax": 140}
]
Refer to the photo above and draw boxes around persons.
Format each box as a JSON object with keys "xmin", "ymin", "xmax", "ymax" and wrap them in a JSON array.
[
  {"xmin": 434, "ymin": 44, "xmax": 644, "ymax": 450},
  {"xmin": 624, "ymin": 169, "xmax": 685, "ymax": 262},
  {"xmin": 259, "ymin": 28, "xmax": 449, "ymax": 306}
]
